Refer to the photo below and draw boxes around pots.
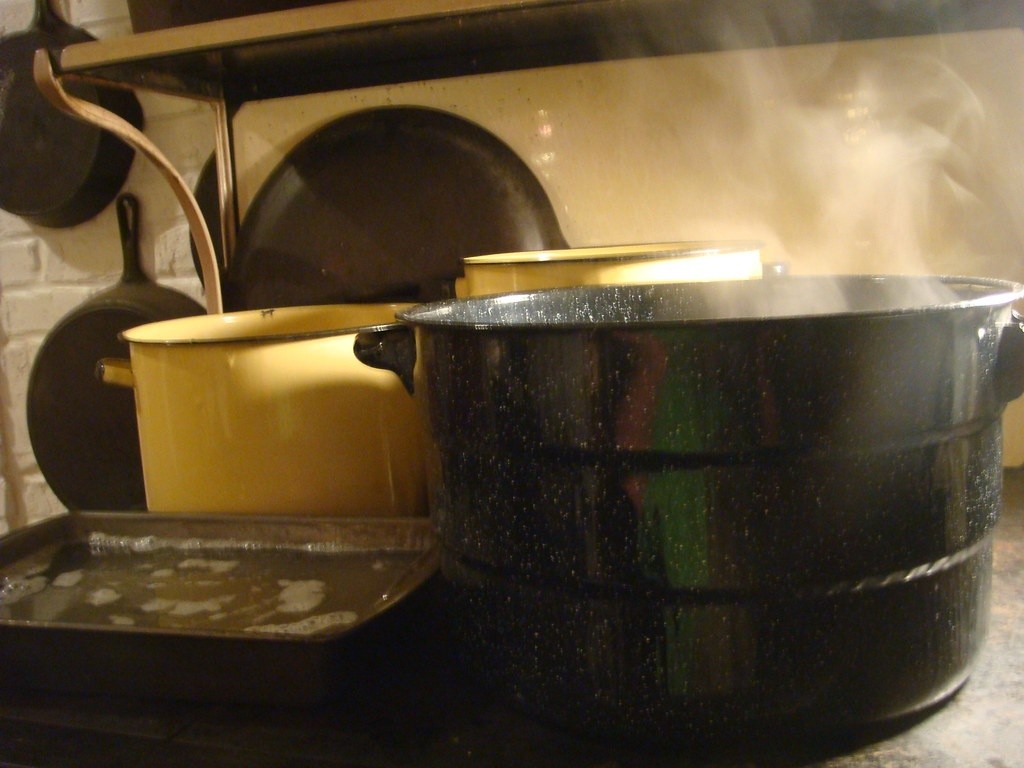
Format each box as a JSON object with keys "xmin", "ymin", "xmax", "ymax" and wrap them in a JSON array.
[
  {"xmin": 96, "ymin": 302, "xmax": 437, "ymax": 519},
  {"xmin": 454, "ymin": 243, "xmax": 765, "ymax": 300},
  {"xmin": 352, "ymin": 271, "xmax": 1024, "ymax": 767},
  {"xmin": 26, "ymin": 193, "xmax": 209, "ymax": 513},
  {"xmin": 0, "ymin": 0, "xmax": 142, "ymax": 229}
]
